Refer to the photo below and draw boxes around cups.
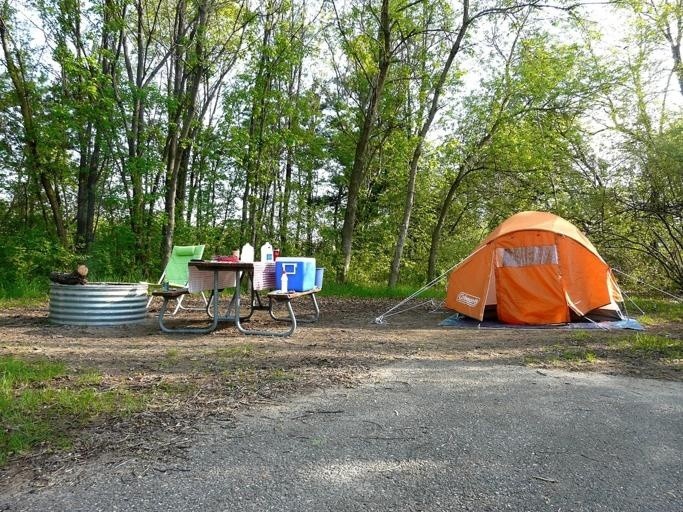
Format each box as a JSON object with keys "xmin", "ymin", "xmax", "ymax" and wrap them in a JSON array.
[{"xmin": 231, "ymin": 251, "xmax": 239, "ymax": 261}]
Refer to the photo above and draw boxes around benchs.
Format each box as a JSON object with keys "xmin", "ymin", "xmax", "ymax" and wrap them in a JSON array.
[
  {"xmin": 152, "ymin": 288, "xmax": 188, "ymax": 334},
  {"xmin": 266, "ymin": 288, "xmax": 323, "ymax": 336}
]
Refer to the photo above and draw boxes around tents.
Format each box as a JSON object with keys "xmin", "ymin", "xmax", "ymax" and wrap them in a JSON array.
[{"xmin": 446, "ymin": 210, "xmax": 624, "ymax": 326}]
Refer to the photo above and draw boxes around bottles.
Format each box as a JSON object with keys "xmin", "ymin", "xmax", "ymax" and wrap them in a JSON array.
[
  {"xmin": 163, "ymin": 274, "xmax": 169, "ymax": 292},
  {"xmin": 273, "ymin": 247, "xmax": 280, "ymax": 260},
  {"xmin": 280, "ymin": 271, "xmax": 289, "ymax": 293}
]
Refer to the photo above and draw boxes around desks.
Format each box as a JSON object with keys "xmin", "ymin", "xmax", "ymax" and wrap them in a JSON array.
[{"xmin": 187, "ymin": 258, "xmax": 275, "ymax": 336}]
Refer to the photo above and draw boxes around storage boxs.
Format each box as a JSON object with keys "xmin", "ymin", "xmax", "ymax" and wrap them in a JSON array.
[
  {"xmin": 274, "ymin": 256, "xmax": 317, "ymax": 292},
  {"xmin": 316, "ymin": 269, "xmax": 324, "ymax": 288}
]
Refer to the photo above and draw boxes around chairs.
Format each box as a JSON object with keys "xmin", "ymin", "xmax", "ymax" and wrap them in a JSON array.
[{"xmin": 146, "ymin": 244, "xmax": 208, "ymax": 316}]
